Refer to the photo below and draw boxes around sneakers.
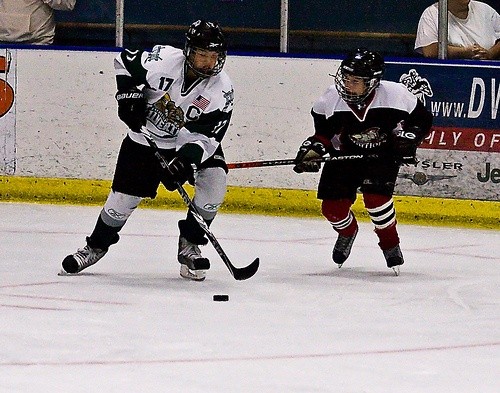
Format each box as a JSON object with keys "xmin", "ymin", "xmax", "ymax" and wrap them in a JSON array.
[
  {"xmin": 177, "ymin": 237, "xmax": 210, "ymax": 282},
  {"xmin": 383, "ymin": 242, "xmax": 404, "ymax": 276},
  {"xmin": 57, "ymin": 234, "xmax": 120, "ymax": 276},
  {"xmin": 332, "ymin": 229, "xmax": 359, "ymax": 269}
]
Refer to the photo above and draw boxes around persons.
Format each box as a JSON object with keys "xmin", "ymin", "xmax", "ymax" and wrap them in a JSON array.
[
  {"xmin": 62, "ymin": 17, "xmax": 235, "ymax": 274},
  {"xmin": 414, "ymin": 0, "xmax": 500, "ymax": 60},
  {"xmin": 0, "ymin": 0, "xmax": 76, "ymax": 46},
  {"xmin": 293, "ymin": 46, "xmax": 433, "ymax": 268}
]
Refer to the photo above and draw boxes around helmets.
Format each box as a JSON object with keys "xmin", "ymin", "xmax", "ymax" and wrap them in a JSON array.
[
  {"xmin": 183, "ymin": 20, "xmax": 226, "ymax": 79},
  {"xmin": 334, "ymin": 49, "xmax": 386, "ymax": 104}
]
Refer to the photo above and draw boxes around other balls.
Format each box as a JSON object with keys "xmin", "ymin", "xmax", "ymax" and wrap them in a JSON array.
[{"xmin": 212, "ymin": 293, "xmax": 229, "ymax": 303}]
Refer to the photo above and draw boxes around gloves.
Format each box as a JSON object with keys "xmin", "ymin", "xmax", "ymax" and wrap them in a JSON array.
[
  {"xmin": 163, "ymin": 142, "xmax": 205, "ymax": 191},
  {"xmin": 115, "ymin": 90, "xmax": 149, "ymax": 134},
  {"xmin": 390, "ymin": 130, "xmax": 420, "ymax": 167},
  {"xmin": 292, "ymin": 136, "xmax": 327, "ymax": 173}
]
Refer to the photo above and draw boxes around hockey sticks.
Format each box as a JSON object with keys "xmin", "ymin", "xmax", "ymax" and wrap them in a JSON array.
[
  {"xmin": 139, "ymin": 124, "xmax": 260, "ymax": 281},
  {"xmin": 228, "ymin": 151, "xmax": 416, "ymax": 170}
]
